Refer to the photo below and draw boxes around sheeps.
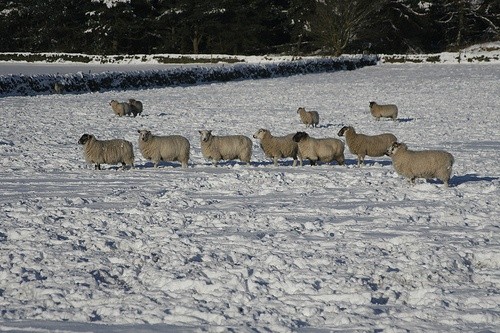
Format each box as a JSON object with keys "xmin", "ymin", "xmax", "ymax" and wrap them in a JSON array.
[
  {"xmin": 297, "ymin": 107, "xmax": 319, "ymax": 128},
  {"xmin": 292, "ymin": 132, "xmax": 345, "ymax": 165},
  {"xmin": 137, "ymin": 129, "xmax": 190, "ymax": 168},
  {"xmin": 337, "ymin": 126, "xmax": 397, "ymax": 167},
  {"xmin": 387, "ymin": 141, "xmax": 455, "ymax": 188},
  {"xmin": 368, "ymin": 101, "xmax": 398, "ymax": 122},
  {"xmin": 253, "ymin": 128, "xmax": 298, "ymax": 166},
  {"xmin": 78, "ymin": 133, "xmax": 135, "ymax": 170},
  {"xmin": 109, "ymin": 99, "xmax": 143, "ymax": 118},
  {"xmin": 197, "ymin": 130, "xmax": 253, "ymax": 167}
]
